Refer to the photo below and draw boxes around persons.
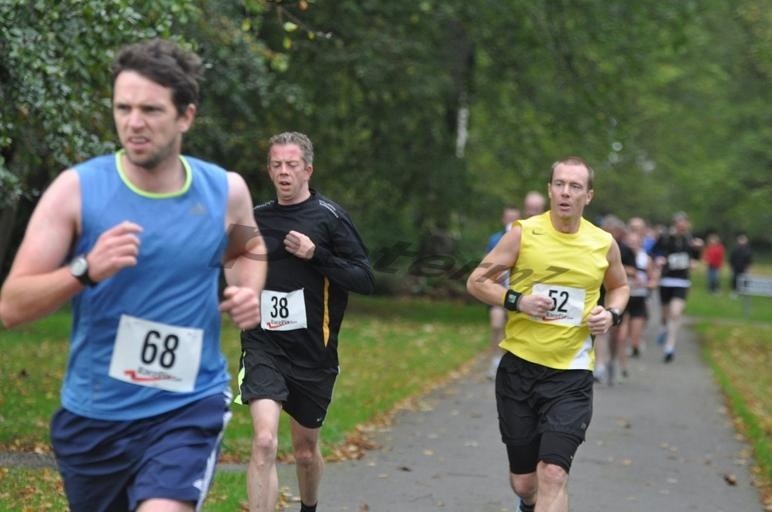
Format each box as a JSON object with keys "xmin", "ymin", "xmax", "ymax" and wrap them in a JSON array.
[
  {"xmin": 465, "ymin": 156, "xmax": 754, "ymax": 512},
  {"xmin": 237, "ymin": 130, "xmax": 376, "ymax": 512},
  {"xmin": 0, "ymin": 38, "xmax": 268, "ymax": 512}
]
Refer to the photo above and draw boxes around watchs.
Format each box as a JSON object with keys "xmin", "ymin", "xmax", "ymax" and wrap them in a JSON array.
[{"xmin": 69, "ymin": 254, "xmax": 98, "ymax": 289}]
